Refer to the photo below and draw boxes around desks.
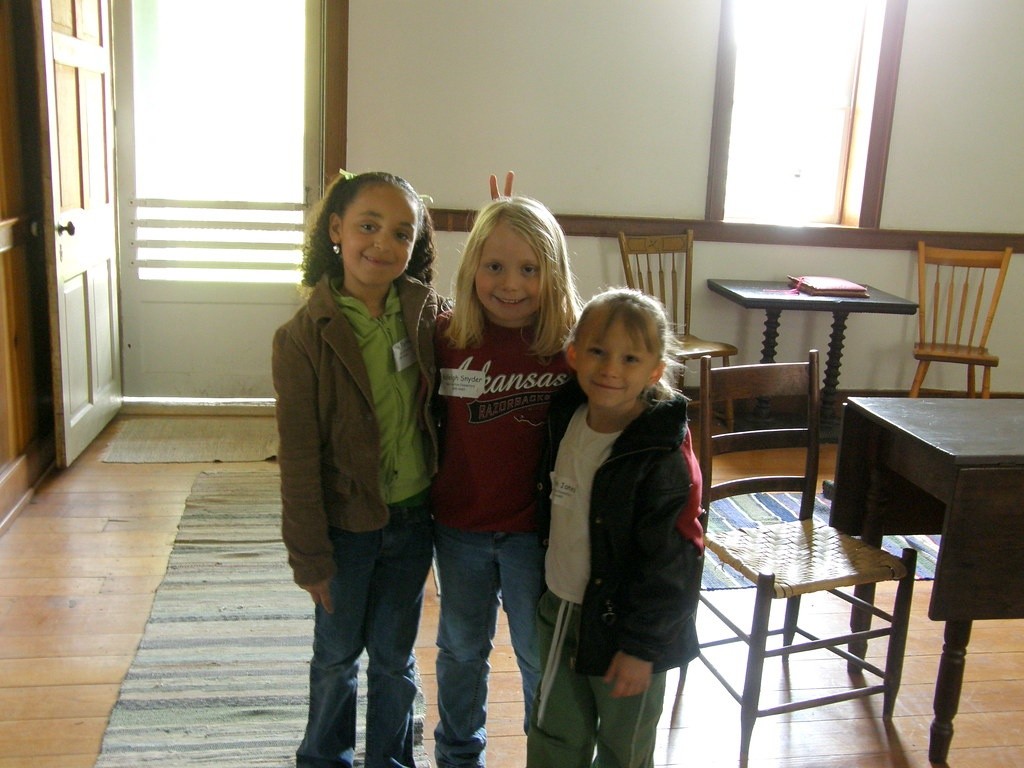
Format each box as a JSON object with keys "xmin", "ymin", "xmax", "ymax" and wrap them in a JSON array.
[
  {"xmin": 706, "ymin": 277, "xmax": 919, "ymax": 442},
  {"xmin": 822, "ymin": 396, "xmax": 1024, "ymax": 761}
]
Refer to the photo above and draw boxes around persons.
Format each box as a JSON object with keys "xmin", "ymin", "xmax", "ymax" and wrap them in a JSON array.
[
  {"xmin": 426, "ymin": 196, "xmax": 582, "ymax": 768},
  {"xmin": 532, "ymin": 289, "xmax": 706, "ymax": 767},
  {"xmin": 271, "ymin": 171, "xmax": 518, "ymax": 768}
]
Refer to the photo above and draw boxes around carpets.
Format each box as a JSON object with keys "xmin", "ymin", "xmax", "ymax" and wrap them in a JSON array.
[
  {"xmin": 100, "ymin": 415, "xmax": 280, "ymax": 463},
  {"xmin": 701, "ymin": 490, "xmax": 943, "ymax": 591},
  {"xmin": 95, "ymin": 470, "xmax": 433, "ymax": 768}
]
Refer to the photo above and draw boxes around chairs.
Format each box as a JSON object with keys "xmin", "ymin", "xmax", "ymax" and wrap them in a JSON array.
[
  {"xmin": 678, "ymin": 348, "xmax": 918, "ymax": 752},
  {"xmin": 620, "ymin": 228, "xmax": 738, "ymax": 434},
  {"xmin": 909, "ymin": 242, "xmax": 1014, "ymax": 400}
]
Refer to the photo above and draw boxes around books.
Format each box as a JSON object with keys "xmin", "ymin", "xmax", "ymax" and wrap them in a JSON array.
[{"xmin": 786, "ymin": 274, "xmax": 872, "ymax": 299}]
